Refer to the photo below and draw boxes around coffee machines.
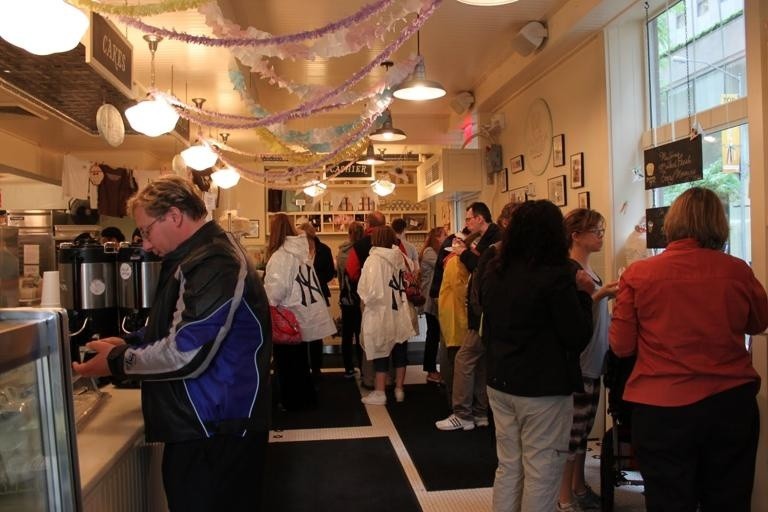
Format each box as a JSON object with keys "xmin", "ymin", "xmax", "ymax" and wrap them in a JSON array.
[{"xmin": 57, "ymin": 232, "xmax": 159, "ymax": 388}]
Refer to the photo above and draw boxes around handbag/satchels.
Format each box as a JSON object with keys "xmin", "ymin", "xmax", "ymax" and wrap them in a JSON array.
[
  {"xmin": 270, "ymin": 305, "xmax": 302, "ymax": 345},
  {"xmin": 404, "ymin": 271, "xmax": 426, "ymax": 306}
]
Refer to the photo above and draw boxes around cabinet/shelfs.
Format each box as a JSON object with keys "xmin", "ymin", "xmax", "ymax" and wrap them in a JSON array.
[{"xmin": 265, "ymin": 164, "xmax": 430, "ymax": 234}]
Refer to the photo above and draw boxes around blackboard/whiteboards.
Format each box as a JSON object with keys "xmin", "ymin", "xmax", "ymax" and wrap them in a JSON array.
[
  {"xmin": 644, "ymin": 133, "xmax": 703, "ymax": 190},
  {"xmin": 646, "ymin": 206, "xmax": 672, "ymax": 249},
  {"xmin": 168, "ymin": 116, "xmax": 191, "ymax": 147},
  {"xmin": 85, "ymin": 8, "xmax": 139, "ymax": 101}
]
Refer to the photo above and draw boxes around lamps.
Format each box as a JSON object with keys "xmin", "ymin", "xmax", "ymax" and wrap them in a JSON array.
[
  {"xmin": 393, "ymin": 13, "xmax": 447, "ymax": 100},
  {"xmin": 181, "ymin": 98, "xmax": 217, "ymax": 171},
  {"xmin": 125, "ymin": 33, "xmax": 178, "ymax": 137},
  {"xmin": 303, "ymin": 153, "xmax": 326, "ymax": 197},
  {"xmin": 356, "ymin": 141, "xmax": 384, "ymax": 165},
  {"xmin": 211, "ymin": 132, "xmax": 240, "ymax": 189},
  {"xmin": 369, "ymin": 61, "xmax": 407, "ymax": 141},
  {"xmin": 371, "ymin": 148, "xmax": 396, "ymax": 196}
]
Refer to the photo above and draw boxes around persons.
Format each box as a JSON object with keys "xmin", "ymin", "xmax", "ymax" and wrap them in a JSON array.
[
  {"xmin": 479, "ymin": 199, "xmax": 621, "ymax": 510},
  {"xmin": 263, "ymin": 199, "xmax": 521, "ymax": 431},
  {"xmin": 73, "ymin": 176, "xmax": 272, "ymax": 512},
  {"xmin": 132, "ymin": 227, "xmax": 143, "ymax": 243},
  {"xmin": 609, "ymin": 187, "xmax": 768, "ymax": 512},
  {"xmin": 101, "ymin": 227, "xmax": 125, "ymax": 246}
]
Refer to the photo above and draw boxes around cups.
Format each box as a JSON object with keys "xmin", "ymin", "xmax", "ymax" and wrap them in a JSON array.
[
  {"xmin": 451, "ymin": 239, "xmax": 462, "ymax": 247},
  {"xmin": 40, "ymin": 270, "xmax": 62, "ymax": 309}
]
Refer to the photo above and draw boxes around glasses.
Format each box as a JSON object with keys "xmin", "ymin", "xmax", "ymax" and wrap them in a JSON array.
[
  {"xmin": 591, "ymin": 228, "xmax": 605, "ymax": 236},
  {"xmin": 140, "ymin": 217, "xmax": 159, "ymax": 241}
]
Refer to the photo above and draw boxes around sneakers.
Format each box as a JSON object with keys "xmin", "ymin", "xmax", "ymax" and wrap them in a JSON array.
[
  {"xmin": 362, "ymin": 391, "xmax": 387, "ymax": 406},
  {"xmin": 435, "ymin": 414, "xmax": 489, "ymax": 431},
  {"xmin": 555, "ymin": 485, "xmax": 600, "ymax": 512},
  {"xmin": 395, "ymin": 392, "xmax": 404, "ymax": 401}
]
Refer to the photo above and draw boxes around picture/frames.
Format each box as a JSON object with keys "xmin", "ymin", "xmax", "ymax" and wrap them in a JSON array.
[
  {"xmin": 497, "ymin": 98, "xmax": 590, "ymax": 211},
  {"xmin": 245, "ymin": 219, "xmax": 259, "ymax": 238}
]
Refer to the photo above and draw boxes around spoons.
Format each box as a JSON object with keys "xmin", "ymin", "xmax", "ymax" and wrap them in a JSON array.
[{"xmin": 616, "ymin": 266, "xmax": 624, "ymax": 284}]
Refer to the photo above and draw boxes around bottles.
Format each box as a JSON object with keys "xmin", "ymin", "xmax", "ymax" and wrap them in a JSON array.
[
  {"xmin": 376, "ymin": 199, "xmax": 433, "ymax": 251},
  {"xmin": 329, "ymin": 201, "xmax": 334, "ymax": 211}
]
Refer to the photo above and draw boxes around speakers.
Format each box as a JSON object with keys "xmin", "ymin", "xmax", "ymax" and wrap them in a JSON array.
[
  {"xmin": 450, "ymin": 91, "xmax": 475, "ymax": 117},
  {"xmin": 509, "ymin": 22, "xmax": 548, "ymax": 58}
]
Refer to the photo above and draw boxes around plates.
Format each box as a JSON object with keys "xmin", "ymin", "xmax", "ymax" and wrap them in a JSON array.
[{"xmin": 444, "ymin": 246, "xmax": 453, "ymax": 253}]
